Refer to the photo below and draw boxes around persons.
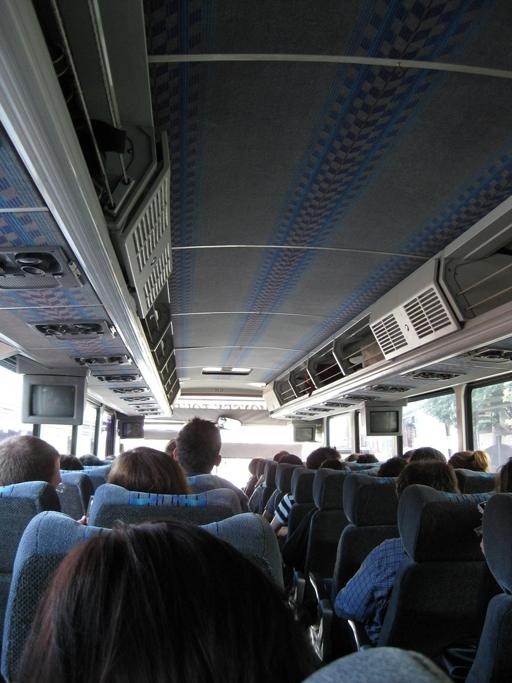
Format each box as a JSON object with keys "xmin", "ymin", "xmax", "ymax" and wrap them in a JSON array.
[
  {"xmin": 0, "ymin": 435, "xmax": 89, "ymax": 524},
  {"xmin": 333, "ymin": 458, "xmax": 462, "ymax": 640},
  {"xmin": 242, "ymin": 445, "xmax": 512, "ymax": 540},
  {"xmin": 105, "ymin": 445, "xmax": 187, "ymax": 494},
  {"xmin": 59, "ymin": 453, "xmax": 84, "ymax": 474},
  {"xmin": 172, "ymin": 417, "xmax": 223, "ymax": 478},
  {"xmin": 21, "ymin": 520, "xmax": 321, "ymax": 682}
]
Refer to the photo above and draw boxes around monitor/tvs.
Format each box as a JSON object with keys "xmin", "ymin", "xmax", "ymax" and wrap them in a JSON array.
[
  {"xmin": 292, "ymin": 422, "xmax": 317, "ymax": 443},
  {"xmin": 365, "ymin": 405, "xmax": 404, "ymax": 437},
  {"xmin": 20, "ymin": 374, "xmax": 85, "ymax": 428},
  {"xmin": 118, "ymin": 419, "xmax": 143, "ymax": 438}
]
